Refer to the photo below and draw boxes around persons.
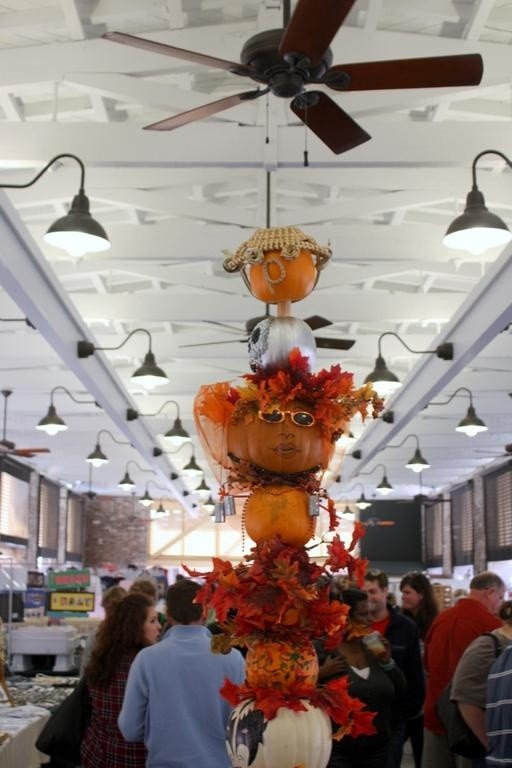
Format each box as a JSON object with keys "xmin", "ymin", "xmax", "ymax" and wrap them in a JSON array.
[
  {"xmin": 36, "ymin": 565, "xmax": 246, "ymax": 767},
  {"xmin": 314, "ymin": 565, "xmax": 512, "ymax": 767}
]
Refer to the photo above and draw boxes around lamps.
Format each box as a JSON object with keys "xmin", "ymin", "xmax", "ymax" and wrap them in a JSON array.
[
  {"xmin": 361, "ymin": 330, "xmax": 456, "ymax": 391},
  {"xmin": 75, "ymin": 327, "xmax": 172, "ymax": 388},
  {"xmin": 441, "ymin": 148, "xmax": 512, "ymax": 256},
  {"xmin": 1, "ymin": 151, "xmax": 112, "ymax": 259},
  {"xmin": 36, "ymin": 385, "xmax": 225, "ymax": 532},
  {"xmin": 330, "ymin": 383, "xmax": 496, "ymax": 527}
]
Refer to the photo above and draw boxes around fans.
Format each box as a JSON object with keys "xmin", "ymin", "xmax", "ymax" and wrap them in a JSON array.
[
  {"xmin": 1, "ymin": 389, "xmax": 51, "ymax": 459},
  {"xmin": 175, "ymin": 169, "xmax": 357, "ymax": 353},
  {"xmin": 98, "ymin": 0, "xmax": 484, "ymax": 168}
]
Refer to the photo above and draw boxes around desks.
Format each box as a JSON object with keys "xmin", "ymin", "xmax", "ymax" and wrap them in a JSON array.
[{"xmin": 0, "ymin": 676, "xmax": 82, "ymax": 768}]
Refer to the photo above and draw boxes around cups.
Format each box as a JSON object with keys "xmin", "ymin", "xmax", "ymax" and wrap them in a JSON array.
[{"xmin": 363, "ymin": 631, "xmax": 388, "ymax": 660}]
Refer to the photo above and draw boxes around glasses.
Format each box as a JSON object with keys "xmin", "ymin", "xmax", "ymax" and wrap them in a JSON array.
[{"xmin": 257, "ymin": 407, "xmax": 319, "ymax": 427}]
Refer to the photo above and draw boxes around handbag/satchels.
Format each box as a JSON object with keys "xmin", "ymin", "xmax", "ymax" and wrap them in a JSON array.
[
  {"xmin": 435, "ymin": 681, "xmax": 485, "ymax": 763},
  {"xmin": 34, "ymin": 672, "xmax": 92, "ymax": 760}
]
